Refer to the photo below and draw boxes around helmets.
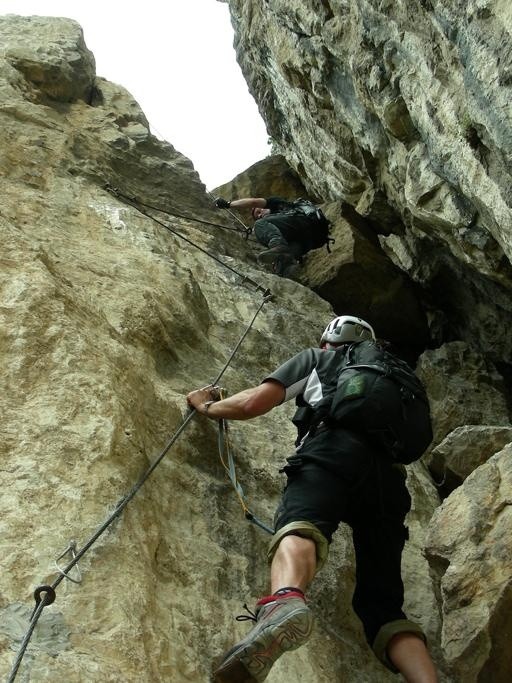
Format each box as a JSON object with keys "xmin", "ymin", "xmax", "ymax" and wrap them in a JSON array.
[{"xmin": 319, "ymin": 316, "xmax": 376, "ymax": 345}]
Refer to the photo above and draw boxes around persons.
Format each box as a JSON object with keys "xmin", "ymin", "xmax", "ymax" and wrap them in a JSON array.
[
  {"xmin": 214, "ymin": 197, "xmax": 318, "ymax": 276},
  {"xmin": 186, "ymin": 312, "xmax": 439, "ymax": 682}
]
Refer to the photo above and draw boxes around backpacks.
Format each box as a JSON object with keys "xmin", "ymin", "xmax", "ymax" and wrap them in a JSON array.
[
  {"xmin": 331, "ymin": 340, "xmax": 433, "ymax": 464},
  {"xmin": 293, "ymin": 197, "xmax": 330, "ymax": 249}
]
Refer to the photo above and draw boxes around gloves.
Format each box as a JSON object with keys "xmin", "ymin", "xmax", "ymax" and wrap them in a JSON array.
[{"xmin": 212, "ymin": 197, "xmax": 230, "ymax": 209}]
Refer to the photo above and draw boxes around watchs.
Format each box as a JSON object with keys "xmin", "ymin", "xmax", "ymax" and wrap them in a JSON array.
[{"xmin": 201, "ymin": 399, "xmax": 216, "ymax": 417}]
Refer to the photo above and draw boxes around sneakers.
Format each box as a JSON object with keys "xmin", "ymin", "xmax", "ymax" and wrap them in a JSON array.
[
  {"xmin": 258, "ymin": 238, "xmax": 289, "ymax": 261},
  {"xmin": 216, "ymin": 587, "xmax": 313, "ymax": 683}
]
